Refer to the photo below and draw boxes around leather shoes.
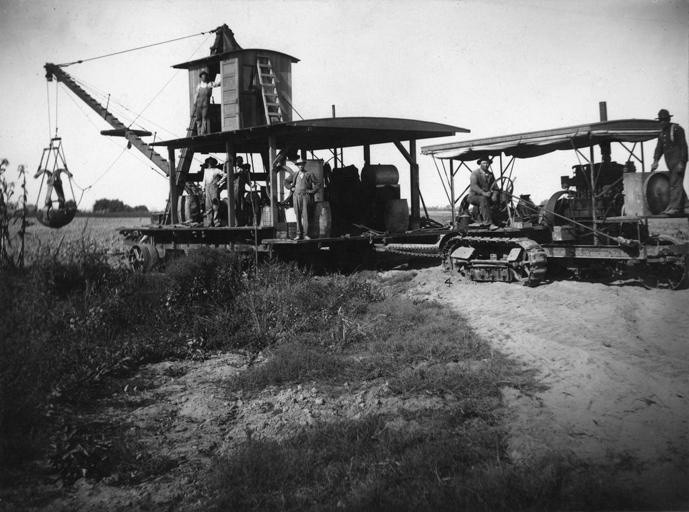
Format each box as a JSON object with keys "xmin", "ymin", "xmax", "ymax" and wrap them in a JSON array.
[
  {"xmin": 662, "ymin": 207, "xmax": 686, "ymax": 215},
  {"xmin": 487, "ymin": 224, "xmax": 499, "ymax": 231},
  {"xmin": 293, "ymin": 235, "xmax": 311, "ymax": 241}
]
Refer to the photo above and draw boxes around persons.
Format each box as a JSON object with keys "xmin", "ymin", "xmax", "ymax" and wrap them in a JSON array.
[
  {"xmin": 203, "ymin": 157, "xmax": 227, "ymax": 227},
  {"xmin": 283, "ymin": 158, "xmax": 321, "ymax": 241},
  {"xmin": 516, "ymin": 193, "xmax": 536, "ymax": 218},
  {"xmin": 232, "ymin": 156, "xmax": 251, "ymax": 212},
  {"xmin": 193, "ymin": 70, "xmax": 221, "ymax": 137},
  {"xmin": 466, "ymin": 156, "xmax": 504, "ymax": 229},
  {"xmin": 650, "ymin": 109, "xmax": 689, "ymax": 215}
]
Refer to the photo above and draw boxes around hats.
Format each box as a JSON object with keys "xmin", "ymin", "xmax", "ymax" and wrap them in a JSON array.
[
  {"xmin": 295, "ymin": 158, "xmax": 307, "ymax": 166},
  {"xmin": 477, "ymin": 155, "xmax": 493, "ymax": 165},
  {"xmin": 199, "ymin": 71, "xmax": 208, "ymax": 78},
  {"xmin": 655, "ymin": 109, "xmax": 674, "ymax": 120},
  {"xmin": 205, "ymin": 157, "xmax": 217, "ymax": 165}
]
